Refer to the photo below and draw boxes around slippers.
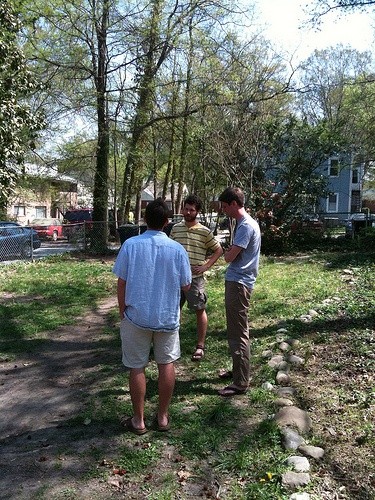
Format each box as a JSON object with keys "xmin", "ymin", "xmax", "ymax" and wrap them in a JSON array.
[
  {"xmin": 121, "ymin": 415, "xmax": 148, "ymax": 435},
  {"xmin": 216, "ymin": 382, "xmax": 255, "ymax": 396},
  {"xmin": 155, "ymin": 413, "xmax": 170, "ymax": 431},
  {"xmin": 218, "ymin": 370, "xmax": 233, "ymax": 379}
]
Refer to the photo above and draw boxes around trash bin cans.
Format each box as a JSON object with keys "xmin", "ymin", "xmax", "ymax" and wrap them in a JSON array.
[
  {"xmin": 348, "ymin": 217, "xmax": 369, "ymax": 241},
  {"xmin": 118, "ymin": 222, "xmax": 174, "ymax": 249}
]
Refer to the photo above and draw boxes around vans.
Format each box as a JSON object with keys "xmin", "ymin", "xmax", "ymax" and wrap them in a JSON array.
[{"xmin": 62, "ymin": 208, "xmax": 134, "ymax": 240}]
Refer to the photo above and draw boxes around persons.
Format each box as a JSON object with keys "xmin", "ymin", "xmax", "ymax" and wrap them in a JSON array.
[
  {"xmin": 113, "ymin": 198, "xmax": 192, "ymax": 435},
  {"xmin": 169, "ymin": 195, "xmax": 223, "ymax": 360},
  {"xmin": 218, "ymin": 187, "xmax": 261, "ymax": 397}
]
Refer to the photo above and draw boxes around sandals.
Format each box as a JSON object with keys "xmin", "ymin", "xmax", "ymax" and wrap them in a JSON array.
[{"xmin": 192, "ymin": 343, "xmax": 204, "ymax": 361}]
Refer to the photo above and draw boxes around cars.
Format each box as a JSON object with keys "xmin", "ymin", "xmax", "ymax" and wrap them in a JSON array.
[
  {"xmin": 0, "ymin": 222, "xmax": 41, "ymax": 259},
  {"xmin": 25, "ymin": 218, "xmax": 66, "ymax": 241},
  {"xmin": 291, "ymin": 213, "xmax": 324, "ymax": 238},
  {"xmin": 196, "ymin": 217, "xmax": 220, "ymax": 236},
  {"xmin": 343, "ymin": 214, "xmax": 375, "ymax": 238}
]
